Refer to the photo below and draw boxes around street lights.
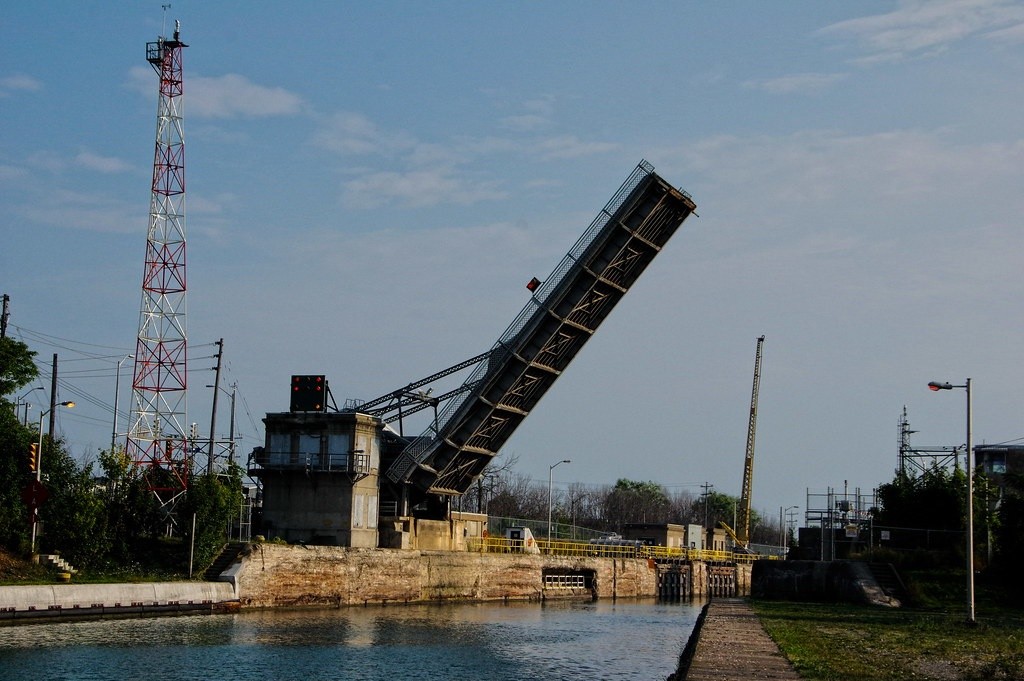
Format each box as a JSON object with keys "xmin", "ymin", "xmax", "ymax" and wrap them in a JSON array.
[
  {"xmin": 927, "ymin": 377, "xmax": 975, "ymax": 622},
  {"xmin": 37, "ymin": 401, "xmax": 76, "ymax": 483},
  {"xmin": 783, "ymin": 505, "xmax": 799, "ymax": 554},
  {"xmin": 16, "ymin": 385, "xmax": 46, "ymax": 423},
  {"xmin": 31, "ymin": 401, "xmax": 76, "ymax": 553},
  {"xmin": 548, "ymin": 460, "xmax": 572, "ymax": 547},
  {"xmin": 206, "ymin": 383, "xmax": 236, "ymax": 466},
  {"xmin": 572, "ymin": 492, "xmax": 592, "ymax": 539}
]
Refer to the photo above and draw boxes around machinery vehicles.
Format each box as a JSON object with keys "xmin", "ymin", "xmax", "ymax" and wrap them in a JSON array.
[{"xmin": 721, "ymin": 332, "xmax": 767, "ymax": 555}]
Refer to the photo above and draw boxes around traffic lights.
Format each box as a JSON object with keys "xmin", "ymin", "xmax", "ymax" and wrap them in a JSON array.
[
  {"xmin": 166, "ymin": 437, "xmax": 172, "ymax": 459},
  {"xmin": 153, "ymin": 418, "xmax": 160, "ymax": 432},
  {"xmin": 30, "ymin": 444, "xmax": 36, "ymax": 471},
  {"xmin": 290, "ymin": 374, "xmax": 326, "ymax": 412},
  {"xmin": 190, "ymin": 423, "xmax": 198, "ymax": 437}
]
